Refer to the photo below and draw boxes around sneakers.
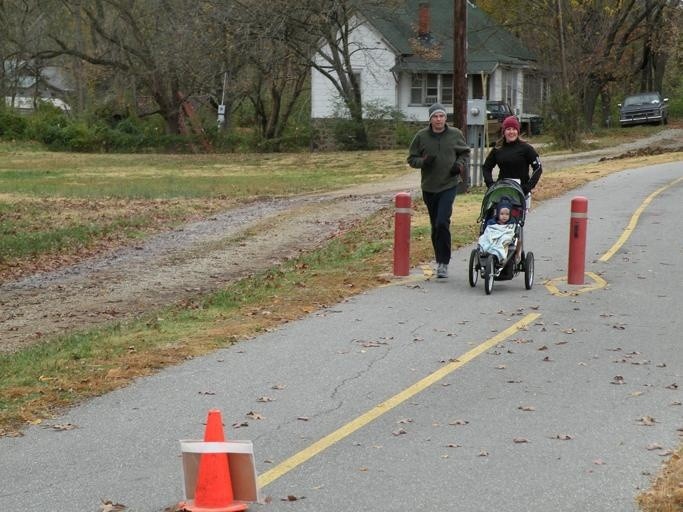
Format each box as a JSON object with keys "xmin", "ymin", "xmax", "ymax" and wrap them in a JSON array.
[{"xmin": 437, "ymin": 264, "xmax": 447, "ymax": 277}]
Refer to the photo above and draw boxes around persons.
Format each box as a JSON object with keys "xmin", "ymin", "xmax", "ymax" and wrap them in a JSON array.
[
  {"xmin": 480, "ymin": 114, "xmax": 543, "ymax": 214},
  {"xmin": 406, "ymin": 103, "xmax": 471, "ymax": 279},
  {"xmin": 479, "ymin": 201, "xmax": 517, "ymax": 276}
]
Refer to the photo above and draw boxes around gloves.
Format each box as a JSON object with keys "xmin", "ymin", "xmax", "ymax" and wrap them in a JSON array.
[
  {"xmin": 423, "ymin": 155, "xmax": 436, "ymax": 165},
  {"xmin": 450, "ymin": 166, "xmax": 460, "ymax": 176}
]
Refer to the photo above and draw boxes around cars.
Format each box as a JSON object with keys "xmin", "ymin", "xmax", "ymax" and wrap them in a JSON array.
[
  {"xmin": 616, "ymin": 90, "xmax": 670, "ymax": 128},
  {"xmin": 485, "ymin": 99, "xmax": 522, "ymax": 127}
]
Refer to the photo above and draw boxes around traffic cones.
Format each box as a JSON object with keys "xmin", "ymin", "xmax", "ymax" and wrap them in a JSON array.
[{"xmin": 181, "ymin": 408, "xmax": 249, "ymax": 511}]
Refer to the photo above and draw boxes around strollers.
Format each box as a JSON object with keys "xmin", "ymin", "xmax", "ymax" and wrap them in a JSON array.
[{"xmin": 467, "ymin": 177, "xmax": 535, "ymax": 295}]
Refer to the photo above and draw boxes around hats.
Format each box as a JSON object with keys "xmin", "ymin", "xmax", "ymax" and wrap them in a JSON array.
[
  {"xmin": 429, "ymin": 103, "xmax": 447, "ymax": 121},
  {"xmin": 503, "ymin": 116, "xmax": 520, "ymax": 136},
  {"xmin": 496, "ymin": 202, "xmax": 512, "ymax": 222}
]
